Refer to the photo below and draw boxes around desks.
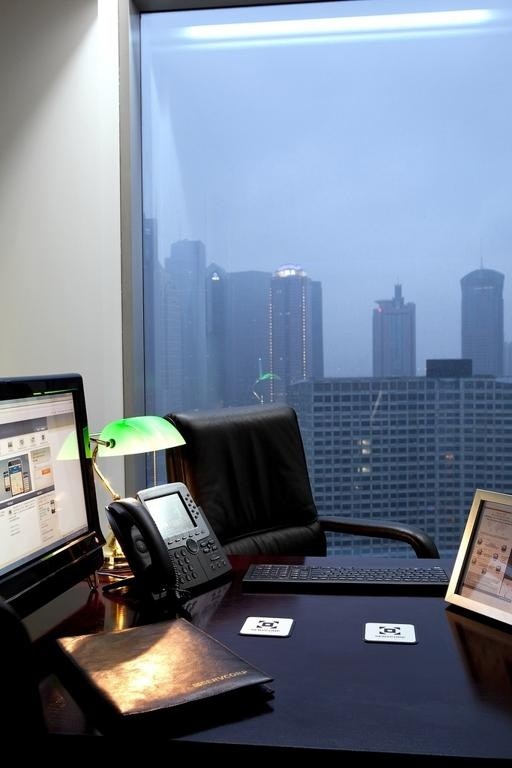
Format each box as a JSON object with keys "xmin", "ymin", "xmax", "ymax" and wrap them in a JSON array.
[{"xmin": 0, "ymin": 552, "xmax": 512, "ymax": 766}]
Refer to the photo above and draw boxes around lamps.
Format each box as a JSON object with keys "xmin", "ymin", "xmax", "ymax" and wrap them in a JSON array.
[{"xmin": 81, "ymin": 416, "xmax": 186, "ymax": 572}]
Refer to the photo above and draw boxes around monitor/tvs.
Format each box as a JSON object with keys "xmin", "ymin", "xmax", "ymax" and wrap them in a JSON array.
[{"xmin": 0, "ymin": 373, "xmax": 107, "ymax": 628}]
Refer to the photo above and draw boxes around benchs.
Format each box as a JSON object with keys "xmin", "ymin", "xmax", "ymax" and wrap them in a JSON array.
[{"xmin": 162, "ymin": 405, "xmax": 441, "ymax": 560}]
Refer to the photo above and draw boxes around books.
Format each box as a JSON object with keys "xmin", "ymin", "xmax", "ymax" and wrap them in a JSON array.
[{"xmin": 56, "ymin": 614, "xmax": 279, "ymax": 737}]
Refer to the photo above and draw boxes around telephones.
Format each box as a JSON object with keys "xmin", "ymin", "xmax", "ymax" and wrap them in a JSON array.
[{"xmin": 105, "ymin": 482, "xmax": 233, "ymax": 601}]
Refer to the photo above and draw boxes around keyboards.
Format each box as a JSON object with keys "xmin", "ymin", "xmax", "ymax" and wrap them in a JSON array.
[{"xmin": 243, "ymin": 562, "xmax": 449, "ymax": 594}]
